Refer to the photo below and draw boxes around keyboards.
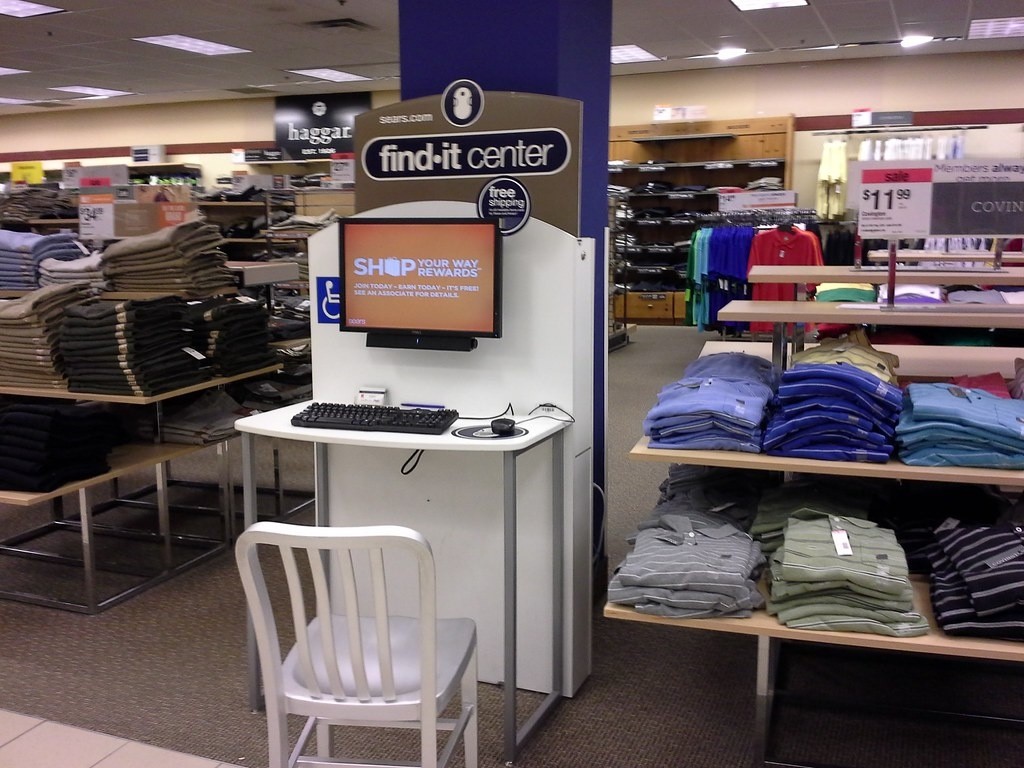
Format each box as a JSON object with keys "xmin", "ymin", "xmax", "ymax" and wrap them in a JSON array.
[{"xmin": 291, "ymin": 402, "xmax": 459, "ymax": 435}]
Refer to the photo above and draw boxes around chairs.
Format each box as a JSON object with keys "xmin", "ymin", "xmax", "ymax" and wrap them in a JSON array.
[{"xmin": 236, "ymin": 521, "xmax": 479, "ymax": 768}]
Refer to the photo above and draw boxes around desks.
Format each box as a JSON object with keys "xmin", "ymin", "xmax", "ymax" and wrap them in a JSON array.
[{"xmin": 235, "ymin": 400, "xmax": 573, "ymax": 768}]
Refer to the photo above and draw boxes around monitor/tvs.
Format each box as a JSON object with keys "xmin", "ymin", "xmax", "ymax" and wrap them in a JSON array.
[{"xmin": 339, "ymin": 217, "xmax": 502, "ymax": 352}]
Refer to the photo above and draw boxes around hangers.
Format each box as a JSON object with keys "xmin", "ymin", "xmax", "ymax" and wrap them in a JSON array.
[{"xmin": 695, "ymin": 207, "xmax": 815, "ymax": 235}]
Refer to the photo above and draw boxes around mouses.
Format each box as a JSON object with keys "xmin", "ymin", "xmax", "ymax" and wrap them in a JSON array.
[{"xmin": 491, "ymin": 418, "xmax": 516, "ymax": 434}]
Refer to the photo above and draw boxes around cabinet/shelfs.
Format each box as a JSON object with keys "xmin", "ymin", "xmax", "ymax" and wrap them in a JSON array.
[
  {"xmin": 607, "ymin": 133, "xmax": 785, "ymax": 270},
  {"xmin": 246, "ymin": 159, "xmax": 357, "ymax": 193},
  {"xmin": 1, "ymin": 162, "xmax": 320, "ymax": 615},
  {"xmin": 614, "ymin": 291, "xmax": 687, "ymax": 326},
  {"xmin": 605, "ymin": 249, "xmax": 1023, "ymax": 768}
]
